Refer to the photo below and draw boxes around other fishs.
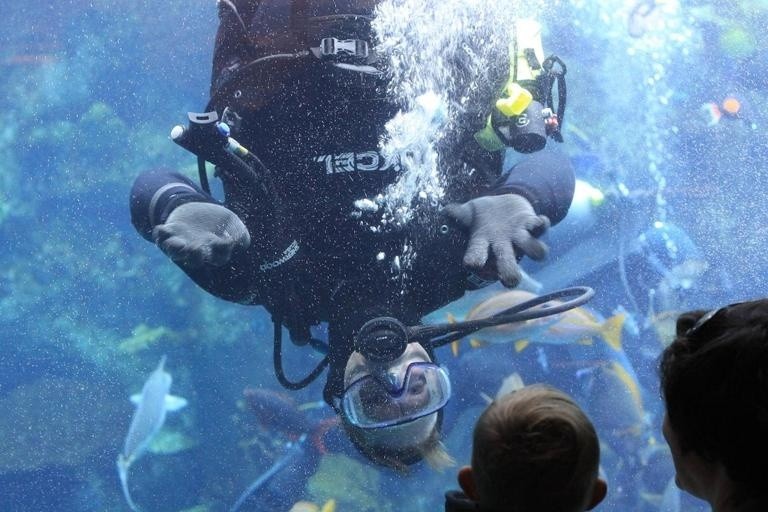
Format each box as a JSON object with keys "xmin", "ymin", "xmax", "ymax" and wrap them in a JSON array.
[{"xmin": 445, "ymin": 256, "xmax": 717, "ymax": 472}]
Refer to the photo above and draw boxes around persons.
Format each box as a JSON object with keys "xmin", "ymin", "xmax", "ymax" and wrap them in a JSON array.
[
  {"xmin": 443, "ymin": 382, "xmax": 608, "ymax": 511},
  {"xmin": 654, "ymin": 298, "xmax": 767, "ymax": 510},
  {"xmin": 129, "ymin": 1, "xmax": 575, "ymax": 471}
]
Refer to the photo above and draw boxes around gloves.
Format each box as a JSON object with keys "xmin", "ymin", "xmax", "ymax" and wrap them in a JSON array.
[
  {"xmin": 151, "ymin": 202, "xmax": 251, "ymax": 266},
  {"xmin": 444, "ymin": 194, "xmax": 551, "ymax": 289}
]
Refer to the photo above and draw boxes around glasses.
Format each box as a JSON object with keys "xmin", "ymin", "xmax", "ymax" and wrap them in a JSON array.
[
  {"xmin": 675, "ymin": 300, "xmax": 746, "ymax": 340},
  {"xmin": 333, "ymin": 362, "xmax": 451, "ymax": 429}
]
